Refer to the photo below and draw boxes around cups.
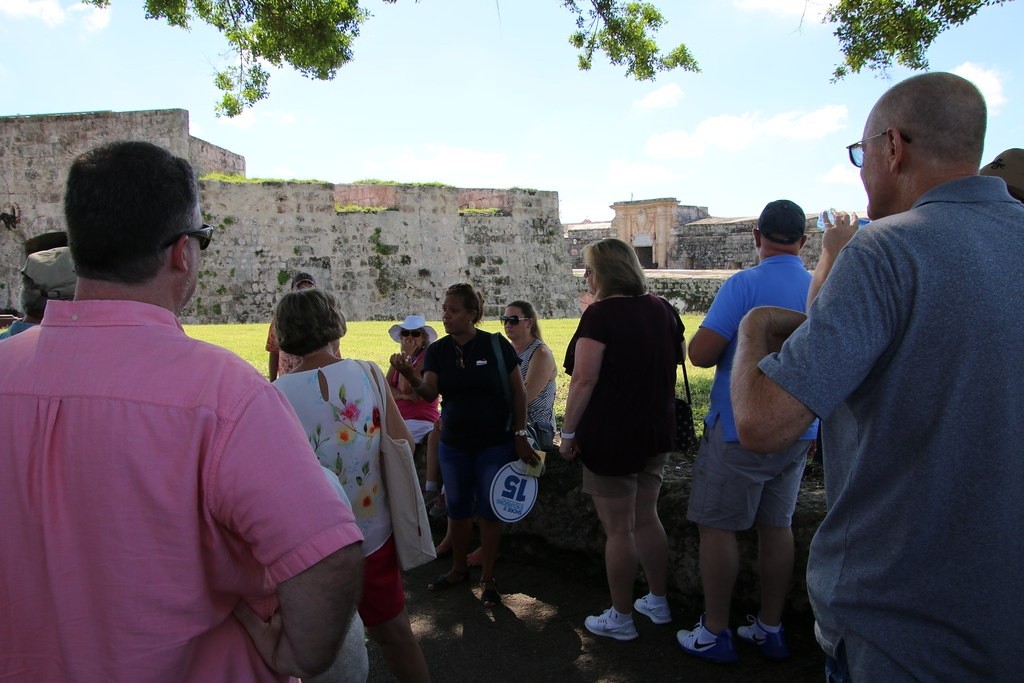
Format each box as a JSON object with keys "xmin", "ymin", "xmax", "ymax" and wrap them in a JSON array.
[{"xmin": 526, "ymin": 450, "xmax": 547, "ymax": 478}]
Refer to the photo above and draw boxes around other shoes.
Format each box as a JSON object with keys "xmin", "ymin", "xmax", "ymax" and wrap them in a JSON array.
[
  {"xmin": 418, "ymin": 488, "xmax": 451, "ymax": 518},
  {"xmin": 433, "ymin": 544, "xmax": 454, "ymax": 555}
]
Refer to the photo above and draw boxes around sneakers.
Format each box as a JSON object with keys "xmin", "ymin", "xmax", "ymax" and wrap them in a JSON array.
[
  {"xmin": 675, "ymin": 614, "xmax": 738, "ymax": 662},
  {"xmin": 736, "ymin": 616, "xmax": 789, "ymax": 658},
  {"xmin": 633, "ymin": 595, "xmax": 673, "ymax": 624},
  {"xmin": 584, "ymin": 610, "xmax": 639, "ymax": 641}
]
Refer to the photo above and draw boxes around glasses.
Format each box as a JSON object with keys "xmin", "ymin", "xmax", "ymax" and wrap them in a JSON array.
[
  {"xmin": 401, "ymin": 330, "xmax": 421, "ymax": 337},
  {"xmin": 846, "ymin": 130, "xmax": 912, "ymax": 168},
  {"xmin": 500, "ymin": 315, "xmax": 529, "ymax": 326},
  {"xmin": 160, "ymin": 223, "xmax": 214, "ymax": 250}
]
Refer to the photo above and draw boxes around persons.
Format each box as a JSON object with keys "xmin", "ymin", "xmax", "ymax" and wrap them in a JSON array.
[
  {"xmin": 265, "ymin": 272, "xmax": 341, "ymax": 382},
  {"xmin": 730, "ymin": 72, "xmax": 1024, "ymax": 683},
  {"xmin": 0, "ymin": 247, "xmax": 77, "ymax": 340},
  {"xmin": 390, "ymin": 283, "xmax": 541, "ymax": 608},
  {"xmin": 386, "ymin": 316, "xmax": 440, "ymax": 443},
  {"xmin": 677, "ymin": 200, "xmax": 819, "ymax": 663},
  {"xmin": 0, "ymin": 141, "xmax": 367, "ymax": 683},
  {"xmin": 559, "ymin": 238, "xmax": 686, "ymax": 640},
  {"xmin": 435, "ymin": 300, "xmax": 557, "ymax": 567},
  {"xmin": 272, "ymin": 288, "xmax": 432, "ymax": 683},
  {"xmin": 422, "ymin": 419, "xmax": 447, "ymax": 516}
]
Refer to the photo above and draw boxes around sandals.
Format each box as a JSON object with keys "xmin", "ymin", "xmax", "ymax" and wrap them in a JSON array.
[
  {"xmin": 479, "ymin": 578, "xmax": 501, "ymax": 609},
  {"xmin": 427, "ymin": 569, "xmax": 470, "ymax": 592}
]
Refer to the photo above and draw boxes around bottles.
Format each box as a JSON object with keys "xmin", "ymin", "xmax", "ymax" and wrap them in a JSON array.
[{"xmin": 817, "ymin": 209, "xmax": 872, "ymax": 232}]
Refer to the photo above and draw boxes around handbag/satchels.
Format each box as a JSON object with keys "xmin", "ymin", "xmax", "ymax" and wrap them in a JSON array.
[
  {"xmin": 660, "ymin": 296, "xmax": 699, "ymax": 454},
  {"xmin": 531, "ymin": 420, "xmax": 555, "ymax": 451},
  {"xmin": 353, "ymin": 359, "xmax": 437, "ymax": 572},
  {"xmin": 491, "ymin": 332, "xmax": 547, "ymax": 480}
]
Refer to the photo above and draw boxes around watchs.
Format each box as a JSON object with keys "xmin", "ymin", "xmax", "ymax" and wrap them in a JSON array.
[{"xmin": 515, "ymin": 430, "xmax": 527, "ymax": 436}]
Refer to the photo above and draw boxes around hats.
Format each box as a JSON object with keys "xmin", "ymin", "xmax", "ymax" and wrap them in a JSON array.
[
  {"xmin": 757, "ymin": 199, "xmax": 806, "ymax": 244},
  {"xmin": 388, "ymin": 316, "xmax": 438, "ymax": 345},
  {"xmin": 291, "ymin": 273, "xmax": 315, "ymax": 289},
  {"xmin": 19, "ymin": 247, "xmax": 79, "ymax": 298},
  {"xmin": 979, "ymin": 148, "xmax": 1024, "ymax": 198}
]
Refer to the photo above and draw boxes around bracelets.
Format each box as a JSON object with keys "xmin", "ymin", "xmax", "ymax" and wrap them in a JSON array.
[{"xmin": 560, "ymin": 427, "xmax": 575, "ymax": 439}]
